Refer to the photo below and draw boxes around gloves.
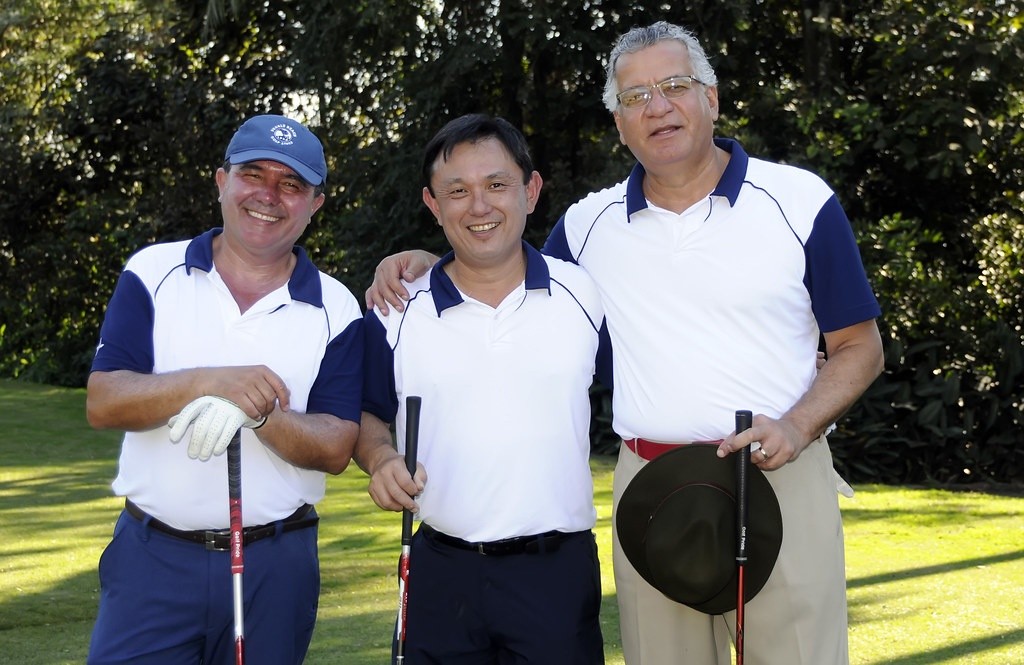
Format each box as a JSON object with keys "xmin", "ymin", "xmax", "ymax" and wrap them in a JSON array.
[{"xmin": 168, "ymin": 396, "xmax": 267, "ymax": 461}]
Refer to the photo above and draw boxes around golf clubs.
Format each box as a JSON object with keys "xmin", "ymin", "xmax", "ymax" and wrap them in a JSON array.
[
  {"xmin": 732, "ymin": 408, "xmax": 754, "ymax": 664},
  {"xmin": 225, "ymin": 427, "xmax": 247, "ymax": 665},
  {"xmin": 394, "ymin": 395, "xmax": 425, "ymax": 664}
]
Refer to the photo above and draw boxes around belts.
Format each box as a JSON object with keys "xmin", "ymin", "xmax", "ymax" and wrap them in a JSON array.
[
  {"xmin": 419, "ymin": 521, "xmax": 591, "ymax": 557},
  {"xmin": 125, "ymin": 497, "xmax": 320, "ymax": 549},
  {"xmin": 624, "ymin": 438, "xmax": 724, "ymax": 461}
]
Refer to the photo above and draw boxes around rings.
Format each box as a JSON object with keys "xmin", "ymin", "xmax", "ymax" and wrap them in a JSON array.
[{"xmin": 759, "ymin": 447, "xmax": 769, "ymax": 461}]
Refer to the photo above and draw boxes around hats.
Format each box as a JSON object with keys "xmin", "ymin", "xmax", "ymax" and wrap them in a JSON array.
[
  {"xmin": 616, "ymin": 444, "xmax": 783, "ymax": 615},
  {"xmin": 225, "ymin": 115, "xmax": 327, "ymax": 187}
]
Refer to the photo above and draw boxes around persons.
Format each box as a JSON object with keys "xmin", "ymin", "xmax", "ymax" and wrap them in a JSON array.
[
  {"xmin": 366, "ymin": 21, "xmax": 887, "ymax": 665},
  {"xmin": 83, "ymin": 113, "xmax": 364, "ymax": 665},
  {"xmin": 352, "ymin": 114, "xmax": 613, "ymax": 665}
]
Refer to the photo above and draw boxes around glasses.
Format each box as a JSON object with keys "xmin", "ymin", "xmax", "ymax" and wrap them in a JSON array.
[{"xmin": 616, "ymin": 76, "xmax": 706, "ymax": 108}]
